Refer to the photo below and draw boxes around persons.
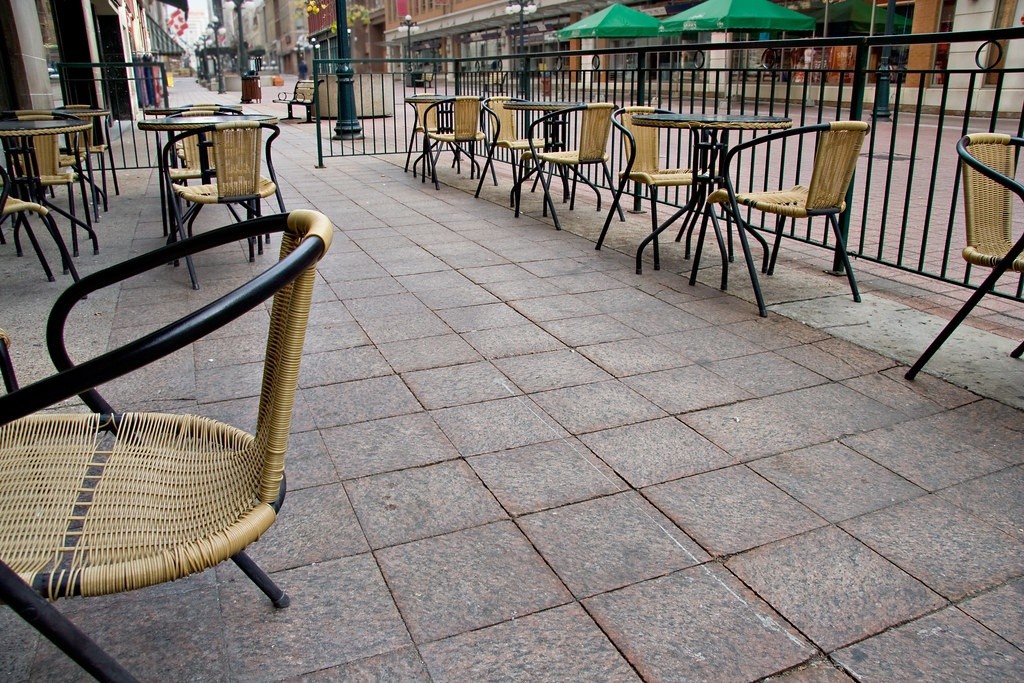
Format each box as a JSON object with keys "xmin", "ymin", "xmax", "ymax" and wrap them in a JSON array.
[
  {"xmin": 297, "ymin": 60, "xmax": 306, "ymax": 78},
  {"xmin": 804, "ymin": 46, "xmax": 816, "ymax": 82}
]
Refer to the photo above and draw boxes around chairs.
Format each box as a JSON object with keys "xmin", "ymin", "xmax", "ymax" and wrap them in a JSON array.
[
  {"xmin": 514, "ymin": 103, "xmax": 625, "ymax": 231},
  {"xmin": 0, "ymin": 104, "xmax": 288, "ymax": 300},
  {"xmin": 905, "ymin": 132, "xmax": 1024, "ymax": 380},
  {"xmin": 0, "ymin": 208, "xmax": 333, "ymax": 683},
  {"xmin": 474, "ymin": 96, "xmax": 571, "ymax": 218},
  {"xmin": 688, "ymin": 121, "xmax": 869, "ymax": 318},
  {"xmin": 421, "ymin": 96, "xmax": 498, "ymax": 190},
  {"xmin": 595, "ymin": 106, "xmax": 719, "ymax": 272},
  {"xmin": 405, "ymin": 92, "xmax": 476, "ymax": 176}
]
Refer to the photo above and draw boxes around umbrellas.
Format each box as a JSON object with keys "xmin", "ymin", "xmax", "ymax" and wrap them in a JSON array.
[
  {"xmin": 809, "ymin": 0, "xmax": 913, "ymax": 31},
  {"xmin": 552, "ymin": 3, "xmax": 661, "ymax": 48},
  {"xmin": 658, "ymin": 0, "xmax": 816, "ymax": 100}
]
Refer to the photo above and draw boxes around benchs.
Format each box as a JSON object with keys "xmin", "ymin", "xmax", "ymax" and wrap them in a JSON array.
[
  {"xmin": 414, "ymin": 73, "xmax": 434, "ymax": 88},
  {"xmin": 480, "ymin": 72, "xmax": 507, "ymax": 93},
  {"xmin": 271, "ymin": 79, "xmax": 325, "ymax": 124}
]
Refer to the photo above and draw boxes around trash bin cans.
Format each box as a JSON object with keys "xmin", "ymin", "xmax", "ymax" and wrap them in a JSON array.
[{"xmin": 241, "ymin": 76, "xmax": 262, "ymax": 100}]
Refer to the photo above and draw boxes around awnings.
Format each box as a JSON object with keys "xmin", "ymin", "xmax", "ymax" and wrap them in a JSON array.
[{"xmin": 144, "ymin": 13, "xmax": 187, "ymax": 56}]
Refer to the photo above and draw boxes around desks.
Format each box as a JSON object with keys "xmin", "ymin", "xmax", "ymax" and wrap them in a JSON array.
[
  {"xmin": 503, "ymin": 101, "xmax": 603, "ymax": 217},
  {"xmin": 0, "ymin": 120, "xmax": 101, "ymax": 257},
  {"xmin": 404, "ymin": 95, "xmax": 485, "ymax": 179},
  {"xmin": 631, "ymin": 114, "xmax": 793, "ymax": 290},
  {"xmin": 48, "ymin": 109, "xmax": 111, "ymax": 212},
  {"xmin": 145, "ymin": 106, "xmax": 243, "ymax": 186},
  {"xmin": 137, "ymin": 115, "xmax": 271, "ymax": 267}
]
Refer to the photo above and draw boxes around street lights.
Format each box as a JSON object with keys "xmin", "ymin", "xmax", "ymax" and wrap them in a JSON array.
[
  {"xmin": 225, "ymin": 0, "xmax": 256, "ymax": 105},
  {"xmin": 505, "ymin": 0, "xmax": 537, "ymax": 95},
  {"xmin": 206, "ymin": 16, "xmax": 228, "ymax": 95},
  {"xmin": 194, "ymin": 32, "xmax": 213, "ymax": 84},
  {"xmin": 308, "ymin": 38, "xmax": 320, "ymax": 60},
  {"xmin": 398, "ymin": 14, "xmax": 419, "ymax": 76},
  {"xmin": 294, "ymin": 41, "xmax": 305, "ymax": 69}
]
